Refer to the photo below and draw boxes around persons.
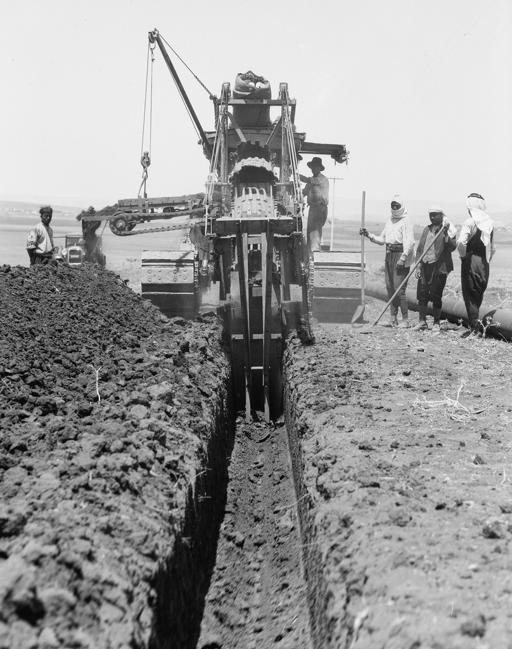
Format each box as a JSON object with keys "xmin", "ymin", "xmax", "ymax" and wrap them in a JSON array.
[
  {"xmin": 359, "ymin": 194, "xmax": 414, "ymax": 332},
  {"xmin": 410, "ymin": 202, "xmax": 457, "ymax": 334},
  {"xmin": 24, "ymin": 205, "xmax": 57, "ymax": 265},
  {"xmin": 296, "ymin": 156, "xmax": 330, "ymax": 255},
  {"xmin": 455, "ymin": 191, "xmax": 497, "ymax": 339}
]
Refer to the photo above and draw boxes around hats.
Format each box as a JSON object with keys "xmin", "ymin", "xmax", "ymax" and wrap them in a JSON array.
[
  {"xmin": 40, "ymin": 208, "xmax": 52, "ymax": 213},
  {"xmin": 307, "ymin": 157, "xmax": 324, "ymax": 171}
]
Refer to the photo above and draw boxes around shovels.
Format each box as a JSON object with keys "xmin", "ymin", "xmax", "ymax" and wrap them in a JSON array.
[
  {"xmin": 360, "ymin": 223, "xmax": 446, "ymax": 333},
  {"xmin": 350, "ymin": 191, "xmax": 366, "ymax": 324}
]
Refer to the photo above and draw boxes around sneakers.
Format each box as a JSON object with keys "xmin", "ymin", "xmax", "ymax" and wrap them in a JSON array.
[
  {"xmin": 398, "ymin": 321, "xmax": 409, "ymax": 328},
  {"xmin": 383, "ymin": 321, "xmax": 397, "ymax": 327},
  {"xmin": 414, "ymin": 323, "xmax": 427, "ymax": 329},
  {"xmin": 460, "ymin": 329, "xmax": 480, "ymax": 339},
  {"xmin": 432, "ymin": 323, "xmax": 440, "ymax": 331}
]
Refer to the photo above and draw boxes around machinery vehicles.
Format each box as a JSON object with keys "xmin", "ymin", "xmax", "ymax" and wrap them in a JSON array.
[
  {"xmin": 80, "ymin": 25, "xmax": 370, "ymax": 418},
  {"xmin": 52, "ymin": 230, "xmax": 110, "ymax": 271}
]
